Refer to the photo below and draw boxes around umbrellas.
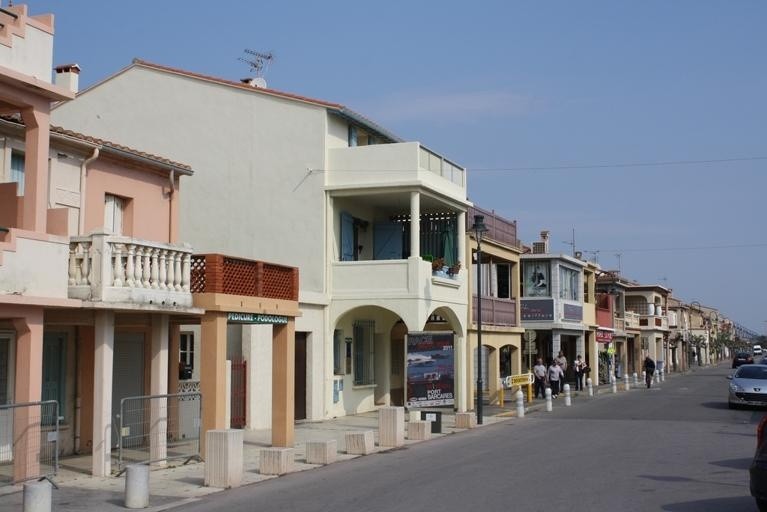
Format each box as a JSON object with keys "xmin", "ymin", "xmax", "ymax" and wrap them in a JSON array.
[{"xmin": 441, "ymin": 220, "xmax": 454, "ymax": 268}]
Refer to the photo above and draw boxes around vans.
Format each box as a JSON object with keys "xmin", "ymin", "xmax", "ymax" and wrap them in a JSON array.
[{"xmin": 753, "ymin": 344, "xmax": 762, "ymax": 354}]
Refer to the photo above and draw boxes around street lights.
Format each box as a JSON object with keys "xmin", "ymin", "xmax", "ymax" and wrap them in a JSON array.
[
  {"xmin": 708, "ymin": 310, "xmax": 757, "ymax": 364},
  {"xmin": 688, "ymin": 301, "xmax": 700, "ymax": 368},
  {"xmin": 469, "ymin": 214, "xmax": 490, "ymax": 425}
]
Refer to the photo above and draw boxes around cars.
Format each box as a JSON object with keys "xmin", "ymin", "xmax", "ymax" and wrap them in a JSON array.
[
  {"xmin": 732, "ymin": 352, "xmax": 754, "ymax": 369},
  {"xmin": 763, "ymin": 349, "xmax": 767, "ymax": 361},
  {"xmin": 726, "ymin": 364, "xmax": 767, "ymax": 410}
]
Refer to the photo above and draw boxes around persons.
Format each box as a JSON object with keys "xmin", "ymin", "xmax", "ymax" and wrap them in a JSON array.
[
  {"xmin": 556, "ymin": 350, "xmax": 568, "ymax": 393},
  {"xmin": 573, "ymin": 354, "xmax": 587, "ymax": 391},
  {"xmin": 534, "ymin": 358, "xmax": 547, "ymax": 398},
  {"xmin": 547, "ymin": 359, "xmax": 564, "ymax": 399},
  {"xmin": 642, "ymin": 354, "xmax": 653, "ymax": 388},
  {"xmin": 534, "ymin": 265, "xmax": 546, "ymax": 287}
]
